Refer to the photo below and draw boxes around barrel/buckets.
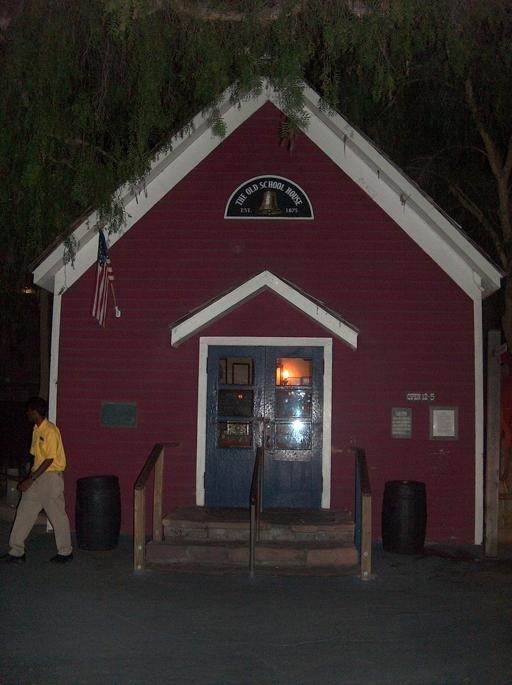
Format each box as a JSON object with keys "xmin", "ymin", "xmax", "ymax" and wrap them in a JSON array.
[
  {"xmin": 74, "ymin": 473, "xmax": 124, "ymax": 552},
  {"xmin": 380, "ymin": 479, "xmax": 429, "ymax": 557}
]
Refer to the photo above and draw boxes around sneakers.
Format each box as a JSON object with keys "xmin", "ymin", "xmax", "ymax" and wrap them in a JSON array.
[
  {"xmin": 0, "ymin": 553, "xmax": 26, "ymax": 565},
  {"xmin": 50, "ymin": 552, "xmax": 73, "ymax": 563}
]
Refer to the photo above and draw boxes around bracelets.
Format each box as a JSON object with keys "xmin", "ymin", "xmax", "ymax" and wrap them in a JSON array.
[{"xmin": 29, "ymin": 475, "xmax": 36, "ymax": 482}]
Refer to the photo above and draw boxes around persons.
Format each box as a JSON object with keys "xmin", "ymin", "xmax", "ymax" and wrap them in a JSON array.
[{"xmin": 0, "ymin": 396, "xmax": 74, "ymax": 564}]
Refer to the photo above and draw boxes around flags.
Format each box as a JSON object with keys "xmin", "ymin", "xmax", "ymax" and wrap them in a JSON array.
[{"xmin": 92, "ymin": 229, "xmax": 115, "ymax": 328}]
